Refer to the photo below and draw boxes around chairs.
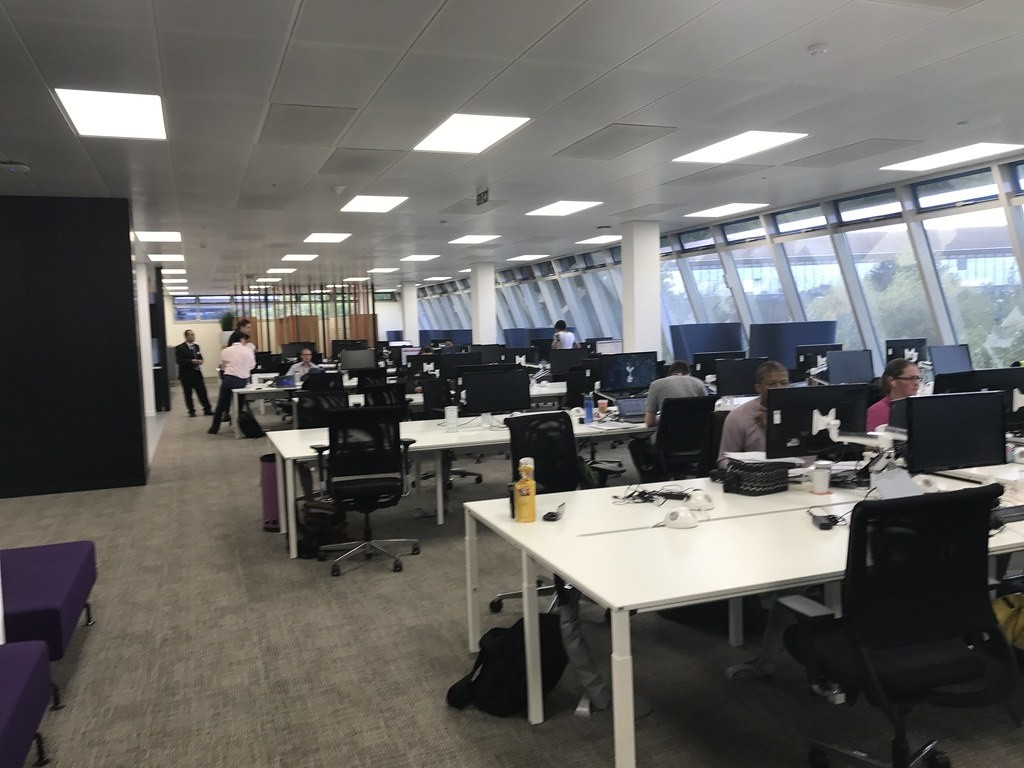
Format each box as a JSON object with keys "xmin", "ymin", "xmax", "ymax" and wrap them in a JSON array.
[
  {"xmin": 762, "ymin": 484, "xmax": 1007, "ymax": 768},
  {"xmin": 278, "ymin": 365, "xmax": 436, "ymax": 487},
  {"xmin": 419, "ymin": 378, "xmax": 481, "ymax": 491},
  {"xmin": 302, "ymin": 406, "xmax": 421, "ymax": 577},
  {"xmin": 641, "ymin": 396, "xmax": 720, "ymax": 484},
  {"xmin": 703, "ymin": 413, "xmax": 733, "ymax": 475},
  {"xmin": 563, "ymin": 367, "xmax": 624, "ymax": 479},
  {"xmin": 488, "ymin": 411, "xmax": 611, "ymax": 625}
]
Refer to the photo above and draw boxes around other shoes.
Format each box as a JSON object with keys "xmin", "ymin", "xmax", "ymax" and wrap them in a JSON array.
[
  {"xmin": 205, "ymin": 410, "xmax": 215, "ymax": 416},
  {"xmin": 190, "ymin": 412, "xmax": 195, "ymax": 417},
  {"xmin": 220, "ymin": 415, "xmax": 230, "ymax": 422},
  {"xmin": 208, "ymin": 427, "xmax": 218, "ymax": 434}
]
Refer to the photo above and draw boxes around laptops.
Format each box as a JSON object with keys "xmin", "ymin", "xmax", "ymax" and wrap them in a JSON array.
[
  {"xmin": 616, "ymin": 397, "xmax": 647, "ymax": 423},
  {"xmin": 275, "ymin": 375, "xmax": 297, "ymax": 388}
]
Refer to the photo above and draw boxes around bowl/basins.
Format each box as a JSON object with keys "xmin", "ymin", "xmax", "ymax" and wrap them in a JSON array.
[{"xmin": 814, "ymin": 460, "xmax": 833, "ymax": 469}]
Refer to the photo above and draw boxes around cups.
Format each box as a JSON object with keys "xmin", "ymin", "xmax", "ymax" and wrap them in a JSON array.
[
  {"xmin": 597, "ymin": 400, "xmax": 608, "ymax": 412},
  {"xmin": 508, "ymin": 481, "xmax": 517, "ymax": 519},
  {"xmin": 809, "ymin": 469, "xmax": 830, "ymax": 494},
  {"xmin": 444, "ymin": 406, "xmax": 458, "ymax": 432}
]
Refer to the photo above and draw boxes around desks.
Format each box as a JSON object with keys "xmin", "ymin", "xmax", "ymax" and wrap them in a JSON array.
[
  {"xmin": 249, "ymin": 373, "xmax": 281, "ymax": 413},
  {"xmin": 231, "ymin": 385, "xmax": 1024, "ymax": 768}
]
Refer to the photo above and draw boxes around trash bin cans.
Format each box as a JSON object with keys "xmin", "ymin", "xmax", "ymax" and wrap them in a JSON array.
[{"xmin": 260, "ymin": 453, "xmax": 298, "ymax": 533}]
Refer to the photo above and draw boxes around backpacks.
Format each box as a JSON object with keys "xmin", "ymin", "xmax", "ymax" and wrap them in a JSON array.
[{"xmin": 446, "ymin": 612, "xmax": 568, "ymax": 719}]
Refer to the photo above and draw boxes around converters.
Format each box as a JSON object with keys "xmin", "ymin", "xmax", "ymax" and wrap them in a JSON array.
[{"xmin": 814, "ymin": 516, "xmax": 833, "ymax": 530}]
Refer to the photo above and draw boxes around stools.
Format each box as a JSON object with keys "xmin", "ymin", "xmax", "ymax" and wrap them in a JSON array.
[{"xmin": 0, "ymin": 539, "xmax": 99, "ymax": 768}]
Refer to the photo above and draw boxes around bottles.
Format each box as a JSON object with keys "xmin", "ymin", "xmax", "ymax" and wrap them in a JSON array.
[
  {"xmin": 294, "ymin": 370, "xmax": 300, "ymax": 383},
  {"xmin": 583, "ymin": 396, "xmax": 594, "ymax": 424},
  {"xmin": 511, "ymin": 457, "xmax": 537, "ymax": 523}
]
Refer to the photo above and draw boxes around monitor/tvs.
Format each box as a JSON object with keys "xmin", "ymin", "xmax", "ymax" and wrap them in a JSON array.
[{"xmin": 251, "ymin": 339, "xmax": 1024, "ymax": 480}]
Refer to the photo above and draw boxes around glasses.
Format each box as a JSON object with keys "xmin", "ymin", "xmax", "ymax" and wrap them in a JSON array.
[{"xmin": 894, "ymin": 373, "xmax": 925, "ymax": 383}]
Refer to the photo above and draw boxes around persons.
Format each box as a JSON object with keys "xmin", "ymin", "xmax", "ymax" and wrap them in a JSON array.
[
  {"xmin": 717, "ymin": 359, "xmax": 819, "ymax": 469},
  {"xmin": 175, "ymin": 328, "xmax": 216, "ymax": 416},
  {"xmin": 552, "ymin": 318, "xmax": 578, "ymax": 349},
  {"xmin": 630, "ymin": 359, "xmax": 708, "ymax": 484},
  {"xmin": 207, "ymin": 319, "xmax": 258, "ymax": 435},
  {"xmin": 286, "ymin": 348, "xmax": 321, "ymax": 382},
  {"xmin": 406, "ymin": 340, "xmax": 461, "ymax": 394},
  {"xmin": 866, "ymin": 356, "xmax": 923, "ymax": 436}
]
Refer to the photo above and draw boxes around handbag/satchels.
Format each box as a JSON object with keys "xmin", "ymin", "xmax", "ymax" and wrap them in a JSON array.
[
  {"xmin": 302, "ymin": 501, "xmax": 344, "ymax": 546},
  {"xmin": 710, "ymin": 459, "xmax": 789, "ymax": 496},
  {"xmin": 237, "ymin": 411, "xmax": 266, "ymax": 439}
]
